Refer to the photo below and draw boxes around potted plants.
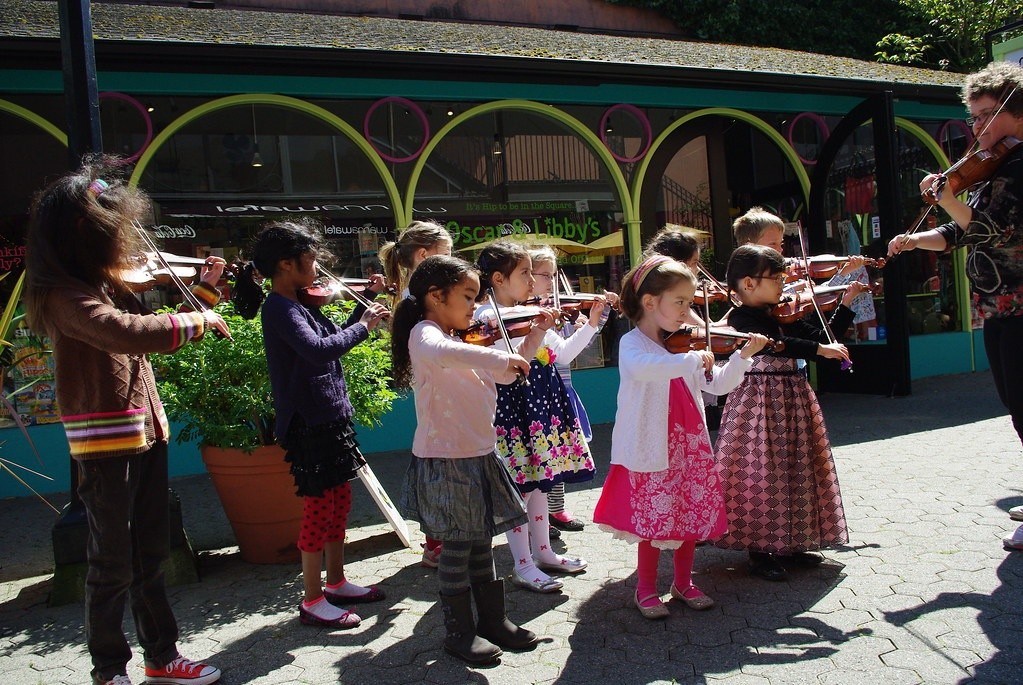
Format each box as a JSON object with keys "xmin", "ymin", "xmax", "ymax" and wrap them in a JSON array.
[{"xmin": 152, "ymin": 299, "xmax": 396, "ymax": 563}]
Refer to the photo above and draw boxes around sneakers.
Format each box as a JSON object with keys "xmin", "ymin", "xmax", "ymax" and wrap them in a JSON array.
[
  {"xmin": 102, "ymin": 674, "xmax": 133, "ymax": 685},
  {"xmin": 145, "ymin": 653, "xmax": 221, "ymax": 685},
  {"xmin": 422, "ymin": 544, "xmax": 442, "ymax": 568}
]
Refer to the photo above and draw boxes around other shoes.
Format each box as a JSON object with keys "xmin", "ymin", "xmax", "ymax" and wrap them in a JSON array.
[
  {"xmin": 324, "ymin": 579, "xmax": 386, "ymax": 607},
  {"xmin": 774, "ymin": 549, "xmax": 821, "ymax": 568},
  {"xmin": 530, "ymin": 554, "xmax": 588, "ymax": 572},
  {"xmin": 634, "ymin": 588, "xmax": 670, "ymax": 619},
  {"xmin": 1002, "ymin": 524, "xmax": 1023, "ymax": 549},
  {"xmin": 746, "ymin": 557, "xmax": 787, "ymax": 579},
  {"xmin": 548, "ymin": 513, "xmax": 584, "ymax": 530},
  {"xmin": 670, "ymin": 581, "xmax": 713, "ymax": 610},
  {"xmin": 298, "ymin": 603, "xmax": 360, "ymax": 629},
  {"xmin": 511, "ymin": 566, "xmax": 563, "ymax": 593},
  {"xmin": 1009, "ymin": 505, "xmax": 1023, "ymax": 520},
  {"xmin": 549, "ymin": 525, "xmax": 561, "ymax": 537}
]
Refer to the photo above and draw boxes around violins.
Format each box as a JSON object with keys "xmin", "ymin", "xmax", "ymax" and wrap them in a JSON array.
[
  {"xmin": 772, "ymin": 253, "xmax": 887, "ymax": 324},
  {"xmin": 453, "ymin": 308, "xmax": 580, "ymax": 348},
  {"xmin": 301, "ymin": 275, "xmax": 399, "ymax": 306},
  {"xmin": 545, "ymin": 291, "xmax": 624, "ymax": 322},
  {"xmin": 109, "ymin": 250, "xmax": 240, "ymax": 294},
  {"xmin": 919, "ymin": 134, "xmax": 1023, "ymax": 207},
  {"xmin": 517, "ymin": 294, "xmax": 601, "ymax": 314},
  {"xmin": 666, "ymin": 279, "xmax": 785, "ymax": 355}
]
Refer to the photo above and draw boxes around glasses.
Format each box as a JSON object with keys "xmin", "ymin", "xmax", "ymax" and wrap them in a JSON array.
[
  {"xmin": 966, "ymin": 108, "xmax": 1010, "ymax": 126},
  {"xmin": 750, "ymin": 276, "xmax": 786, "ymax": 284},
  {"xmin": 532, "ymin": 272, "xmax": 557, "ymax": 281}
]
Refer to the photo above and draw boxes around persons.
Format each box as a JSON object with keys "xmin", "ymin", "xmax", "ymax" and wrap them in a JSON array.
[
  {"xmin": 592, "ymin": 209, "xmax": 869, "ymax": 621},
  {"xmin": 888, "ymin": 60, "xmax": 1023, "ymax": 550},
  {"xmin": 380, "ymin": 222, "xmax": 618, "ymax": 664},
  {"xmin": 232, "ymin": 223, "xmax": 392, "ymax": 630},
  {"xmin": 23, "ymin": 172, "xmax": 230, "ymax": 685}
]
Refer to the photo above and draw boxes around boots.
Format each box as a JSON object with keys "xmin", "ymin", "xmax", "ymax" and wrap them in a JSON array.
[
  {"xmin": 439, "ymin": 591, "xmax": 503, "ymax": 664},
  {"xmin": 471, "ymin": 580, "xmax": 538, "ymax": 650}
]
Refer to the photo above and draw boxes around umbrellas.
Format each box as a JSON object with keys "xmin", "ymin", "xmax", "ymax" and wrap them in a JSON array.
[
  {"xmin": 458, "ymin": 234, "xmax": 592, "ymax": 256},
  {"xmin": 586, "ymin": 222, "xmax": 712, "ymax": 256}
]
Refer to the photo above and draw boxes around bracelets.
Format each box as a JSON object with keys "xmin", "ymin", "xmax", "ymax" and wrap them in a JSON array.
[{"xmin": 201, "ymin": 314, "xmax": 208, "ymax": 329}]
